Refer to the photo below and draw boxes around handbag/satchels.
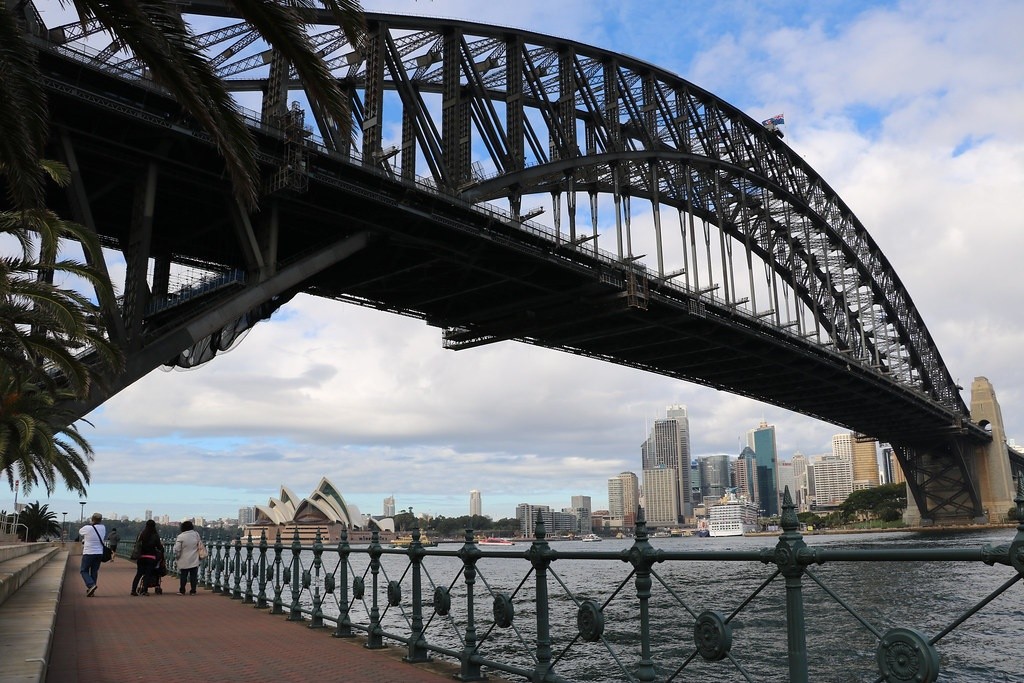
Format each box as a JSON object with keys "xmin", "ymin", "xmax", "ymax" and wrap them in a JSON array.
[
  {"xmin": 197, "ymin": 532, "xmax": 207, "ymax": 559},
  {"xmin": 102, "ymin": 546, "xmax": 112, "ymax": 562},
  {"xmin": 130, "ymin": 542, "xmax": 142, "ymax": 560}
]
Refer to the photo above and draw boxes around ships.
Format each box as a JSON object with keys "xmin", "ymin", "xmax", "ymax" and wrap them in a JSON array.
[{"xmin": 706, "ymin": 487, "xmax": 763, "ymax": 538}]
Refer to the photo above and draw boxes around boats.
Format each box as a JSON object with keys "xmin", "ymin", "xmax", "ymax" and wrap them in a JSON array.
[
  {"xmin": 477, "ymin": 536, "xmax": 515, "ymax": 546},
  {"xmin": 581, "ymin": 533, "xmax": 603, "ymax": 542},
  {"xmin": 389, "ymin": 526, "xmax": 438, "ymax": 549},
  {"xmin": 647, "ymin": 531, "xmax": 672, "ymax": 539}
]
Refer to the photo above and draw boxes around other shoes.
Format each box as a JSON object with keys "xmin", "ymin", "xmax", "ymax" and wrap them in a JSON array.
[
  {"xmin": 177, "ymin": 592, "xmax": 185, "ymax": 595},
  {"xmin": 191, "ymin": 592, "xmax": 196, "ymax": 595},
  {"xmin": 131, "ymin": 592, "xmax": 138, "ymax": 596},
  {"xmin": 87, "ymin": 584, "xmax": 97, "ymax": 596},
  {"xmin": 141, "ymin": 590, "xmax": 149, "ymax": 596}
]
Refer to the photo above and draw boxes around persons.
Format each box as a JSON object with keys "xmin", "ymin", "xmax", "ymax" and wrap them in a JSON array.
[
  {"xmin": 107, "ymin": 528, "xmax": 121, "ymax": 563},
  {"xmin": 174, "ymin": 521, "xmax": 202, "ymax": 597},
  {"xmin": 131, "ymin": 520, "xmax": 165, "ymax": 596},
  {"xmin": 79, "ymin": 513, "xmax": 106, "ymax": 598}
]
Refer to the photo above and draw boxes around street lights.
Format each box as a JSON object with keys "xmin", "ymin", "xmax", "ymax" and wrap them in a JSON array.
[
  {"xmin": 79, "ymin": 501, "xmax": 87, "ymax": 542},
  {"xmin": 62, "ymin": 512, "xmax": 68, "ymax": 543}
]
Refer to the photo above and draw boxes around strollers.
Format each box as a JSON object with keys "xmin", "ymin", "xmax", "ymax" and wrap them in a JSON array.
[{"xmin": 137, "ymin": 556, "xmax": 169, "ymax": 596}]
